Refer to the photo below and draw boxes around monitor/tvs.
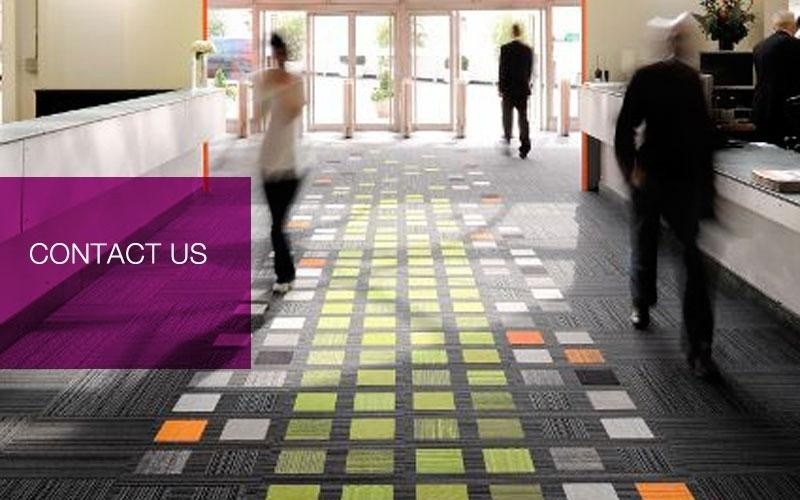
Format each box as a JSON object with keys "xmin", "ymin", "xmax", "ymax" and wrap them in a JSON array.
[{"xmin": 699, "ymin": 51, "xmax": 758, "ymax": 90}]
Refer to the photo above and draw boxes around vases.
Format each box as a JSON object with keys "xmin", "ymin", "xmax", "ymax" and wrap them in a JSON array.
[
  {"xmin": 719, "ymin": 38, "xmax": 733, "ymax": 50},
  {"xmin": 192, "ymin": 53, "xmax": 208, "ymax": 88}
]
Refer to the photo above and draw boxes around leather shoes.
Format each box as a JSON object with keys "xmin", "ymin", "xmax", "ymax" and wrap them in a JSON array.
[
  {"xmin": 628, "ymin": 306, "xmax": 651, "ymax": 329},
  {"xmin": 688, "ymin": 351, "xmax": 721, "ymax": 383}
]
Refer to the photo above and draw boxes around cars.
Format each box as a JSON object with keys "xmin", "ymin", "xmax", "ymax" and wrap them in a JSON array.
[{"xmin": 206, "ymin": 38, "xmax": 253, "ymax": 79}]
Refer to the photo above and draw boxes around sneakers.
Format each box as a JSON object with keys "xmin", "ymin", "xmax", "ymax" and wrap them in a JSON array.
[{"xmin": 273, "ymin": 280, "xmax": 295, "ymax": 294}]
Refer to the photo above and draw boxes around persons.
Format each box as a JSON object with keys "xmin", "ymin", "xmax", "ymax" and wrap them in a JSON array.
[
  {"xmin": 498, "ymin": 23, "xmax": 535, "ymax": 160},
  {"xmin": 609, "ymin": 9, "xmax": 722, "ymax": 378},
  {"xmin": 749, "ymin": 9, "xmax": 800, "ymax": 153},
  {"xmin": 246, "ymin": 31, "xmax": 310, "ymax": 294}
]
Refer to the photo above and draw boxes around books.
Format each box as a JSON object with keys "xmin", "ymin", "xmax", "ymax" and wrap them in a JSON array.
[{"xmin": 751, "ymin": 168, "xmax": 800, "ymax": 194}]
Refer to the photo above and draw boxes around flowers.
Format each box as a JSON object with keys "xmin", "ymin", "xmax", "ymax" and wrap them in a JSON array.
[
  {"xmin": 188, "ymin": 39, "xmax": 216, "ymax": 60},
  {"xmin": 690, "ymin": 0, "xmax": 755, "ymax": 44}
]
